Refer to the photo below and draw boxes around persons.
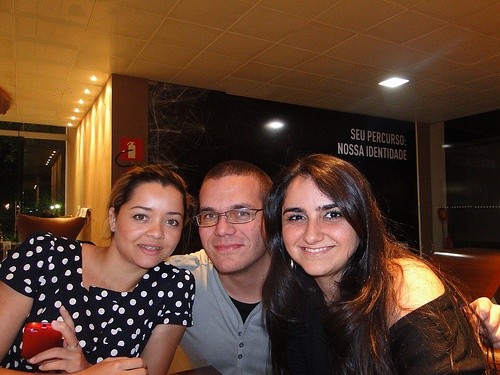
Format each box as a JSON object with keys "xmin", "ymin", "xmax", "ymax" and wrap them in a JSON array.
[
  {"xmin": 165, "ymin": 160, "xmax": 500, "ymax": 375},
  {"xmin": 261, "ymin": 154, "xmax": 496, "ymax": 375},
  {"xmin": 0, "ymin": 164, "xmax": 196, "ymax": 375}
]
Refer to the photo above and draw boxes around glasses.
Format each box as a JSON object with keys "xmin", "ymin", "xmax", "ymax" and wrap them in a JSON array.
[{"xmin": 192, "ymin": 208, "xmax": 264, "ymax": 228}]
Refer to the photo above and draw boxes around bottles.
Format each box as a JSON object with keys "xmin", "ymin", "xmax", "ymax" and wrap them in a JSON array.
[{"xmin": 0, "ymin": 236, "xmax": 4, "ymax": 260}]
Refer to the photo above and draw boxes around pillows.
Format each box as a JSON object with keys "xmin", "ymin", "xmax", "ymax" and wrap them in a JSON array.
[{"xmin": 15, "ymin": 213, "xmax": 86, "ymax": 242}]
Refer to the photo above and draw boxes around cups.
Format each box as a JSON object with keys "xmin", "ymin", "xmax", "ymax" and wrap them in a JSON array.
[{"xmin": 3, "ymin": 241, "xmax": 11, "ymax": 250}]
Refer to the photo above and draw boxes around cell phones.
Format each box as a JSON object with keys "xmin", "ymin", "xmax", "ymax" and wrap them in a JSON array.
[{"xmin": 21, "ymin": 322, "xmax": 62, "ymax": 364}]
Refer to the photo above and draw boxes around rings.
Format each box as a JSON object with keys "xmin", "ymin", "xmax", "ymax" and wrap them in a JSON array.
[{"xmin": 66, "ymin": 340, "xmax": 79, "ymax": 348}]
